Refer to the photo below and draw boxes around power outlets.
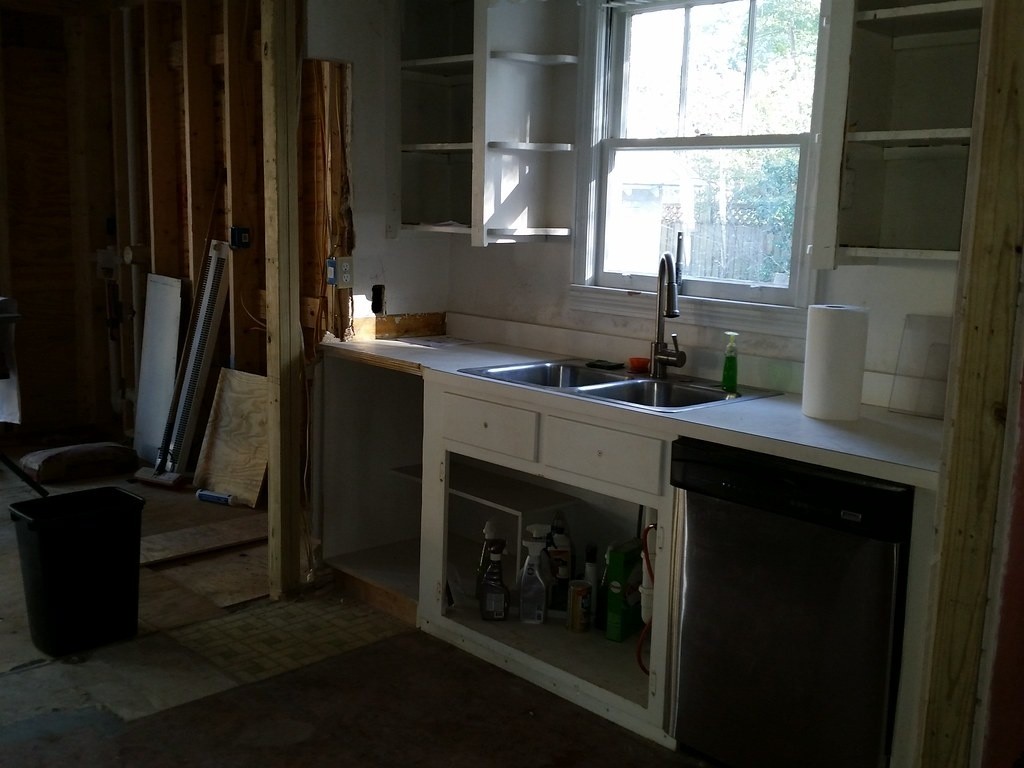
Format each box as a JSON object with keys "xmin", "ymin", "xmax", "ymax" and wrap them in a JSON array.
[{"xmin": 335, "ymin": 257, "xmax": 354, "ymax": 289}]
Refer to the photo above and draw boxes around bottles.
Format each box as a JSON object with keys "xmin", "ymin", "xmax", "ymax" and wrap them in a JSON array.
[{"xmin": 195, "ymin": 489, "xmax": 239, "ymax": 506}]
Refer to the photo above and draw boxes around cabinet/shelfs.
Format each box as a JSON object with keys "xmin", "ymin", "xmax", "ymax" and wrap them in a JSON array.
[
  {"xmin": 398, "ymin": 0, "xmax": 579, "ymax": 248},
  {"xmin": 321, "ymin": 353, "xmax": 687, "ymax": 753},
  {"xmin": 809, "ymin": 0, "xmax": 992, "ymax": 270}
]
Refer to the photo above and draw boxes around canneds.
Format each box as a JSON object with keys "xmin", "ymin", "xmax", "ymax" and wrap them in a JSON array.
[{"xmin": 567, "ymin": 580, "xmax": 592, "ymax": 633}]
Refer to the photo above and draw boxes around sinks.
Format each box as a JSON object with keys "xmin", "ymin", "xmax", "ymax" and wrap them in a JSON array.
[
  {"xmin": 576, "ymin": 373, "xmax": 785, "ymax": 414},
  {"xmin": 455, "ymin": 356, "xmax": 637, "ymax": 394}
]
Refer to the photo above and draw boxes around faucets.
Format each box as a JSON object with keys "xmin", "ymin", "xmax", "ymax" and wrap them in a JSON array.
[{"xmin": 648, "ymin": 250, "xmax": 682, "ymax": 379}]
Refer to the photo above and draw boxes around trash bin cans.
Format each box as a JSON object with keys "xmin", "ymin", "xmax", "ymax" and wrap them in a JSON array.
[{"xmin": 9, "ymin": 487, "xmax": 145, "ymax": 659}]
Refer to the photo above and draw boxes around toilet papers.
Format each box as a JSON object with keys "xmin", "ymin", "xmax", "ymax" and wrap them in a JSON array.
[{"xmin": 800, "ymin": 304, "xmax": 871, "ymax": 422}]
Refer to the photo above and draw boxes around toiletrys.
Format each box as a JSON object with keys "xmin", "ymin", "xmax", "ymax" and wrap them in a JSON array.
[{"xmin": 721, "ymin": 331, "xmax": 739, "ymax": 393}]
